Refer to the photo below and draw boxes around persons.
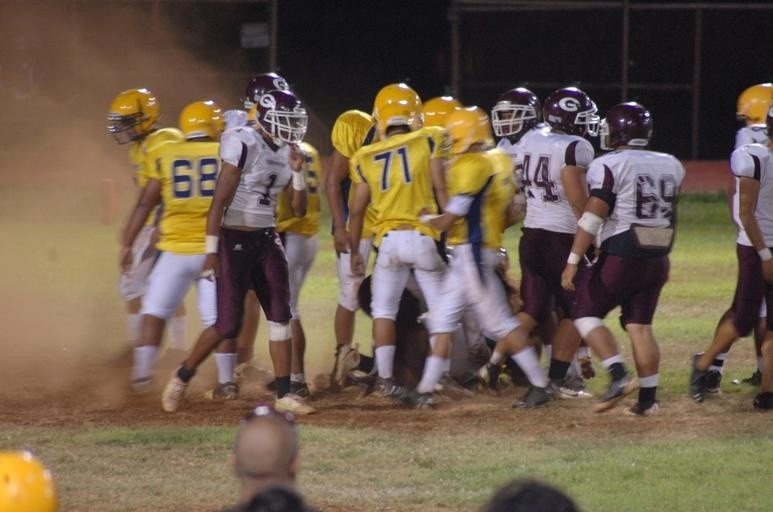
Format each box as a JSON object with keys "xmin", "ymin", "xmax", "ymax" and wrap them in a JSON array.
[
  {"xmin": 709, "ymin": 79, "xmax": 773, "ymax": 400},
  {"xmin": 105, "ymin": 70, "xmax": 686, "ymax": 417},
  {"xmin": 691, "ymin": 104, "xmax": 772, "ymax": 414},
  {"xmin": 219, "ymin": 416, "xmax": 304, "ymax": 511}
]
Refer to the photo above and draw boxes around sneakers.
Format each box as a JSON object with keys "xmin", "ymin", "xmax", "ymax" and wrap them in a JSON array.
[
  {"xmin": 161, "ymin": 360, "xmax": 316, "ymax": 415},
  {"xmin": 332, "ymin": 342, "xmax": 660, "ymax": 416},
  {"xmin": 690, "ymin": 350, "xmax": 773, "ymax": 411}
]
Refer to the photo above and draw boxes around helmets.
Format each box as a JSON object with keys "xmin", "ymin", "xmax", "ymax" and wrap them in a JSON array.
[
  {"xmin": 106, "ymin": 73, "xmax": 310, "ymax": 147},
  {"xmin": 736, "ymin": 82, "xmax": 773, "ymax": 126},
  {"xmin": 373, "ymin": 82, "xmax": 654, "ymax": 155}
]
